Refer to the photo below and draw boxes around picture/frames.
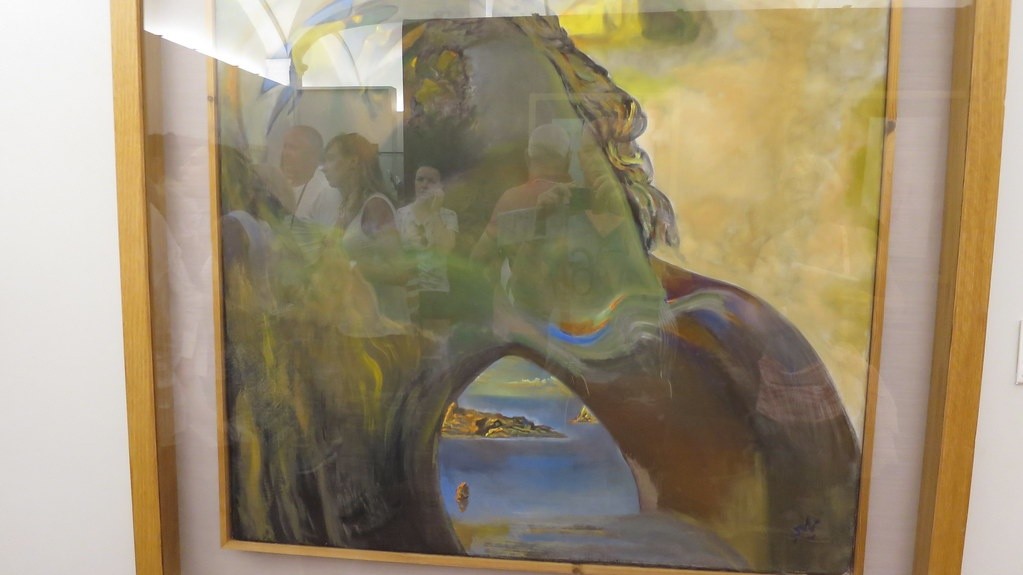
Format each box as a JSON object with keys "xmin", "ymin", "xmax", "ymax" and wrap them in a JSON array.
[{"xmin": 205, "ymin": 1, "xmax": 899, "ymax": 574}]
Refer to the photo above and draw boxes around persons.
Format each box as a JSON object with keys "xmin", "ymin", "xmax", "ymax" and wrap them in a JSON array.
[{"xmin": 219, "ymin": 125, "xmax": 659, "ymax": 342}]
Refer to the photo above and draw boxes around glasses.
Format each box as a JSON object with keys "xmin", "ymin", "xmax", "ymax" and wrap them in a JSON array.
[{"xmin": 415, "ymin": 224, "xmax": 429, "ymax": 247}]
